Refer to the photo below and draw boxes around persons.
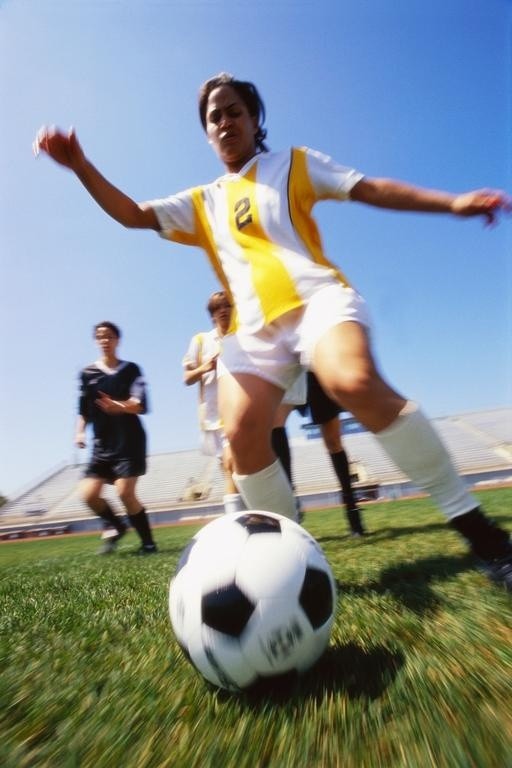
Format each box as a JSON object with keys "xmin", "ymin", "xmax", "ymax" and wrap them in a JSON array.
[
  {"xmin": 182, "ymin": 292, "xmax": 248, "ymax": 514},
  {"xmin": 272, "ymin": 371, "xmax": 363, "ymax": 537},
  {"xmin": 75, "ymin": 322, "xmax": 156, "ymax": 555},
  {"xmin": 33, "ymin": 75, "xmax": 512, "ymax": 591}
]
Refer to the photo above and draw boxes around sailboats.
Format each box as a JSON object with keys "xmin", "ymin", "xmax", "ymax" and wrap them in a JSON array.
[{"xmin": 169, "ymin": 510, "xmax": 336, "ymax": 692}]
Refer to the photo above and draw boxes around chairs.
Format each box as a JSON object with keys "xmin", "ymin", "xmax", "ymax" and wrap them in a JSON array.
[
  {"xmin": 486, "ymin": 554, "xmax": 512, "ymax": 587},
  {"xmin": 99, "ymin": 520, "xmax": 129, "ymax": 542}
]
